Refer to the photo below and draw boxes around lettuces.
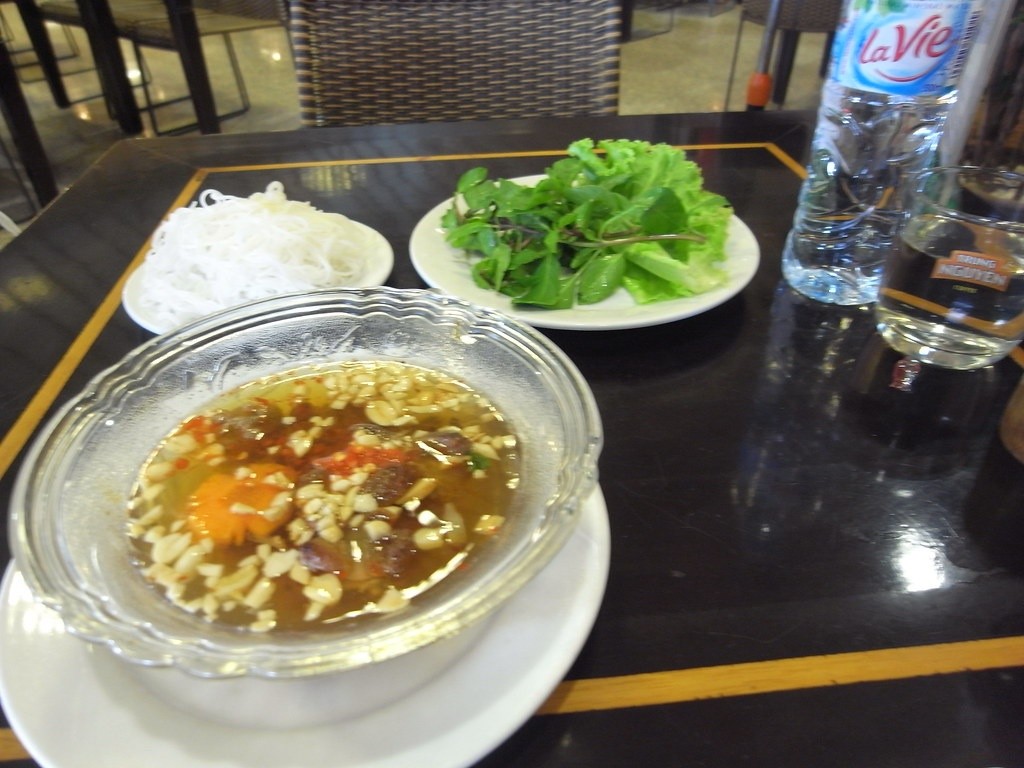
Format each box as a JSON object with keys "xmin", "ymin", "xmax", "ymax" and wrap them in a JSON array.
[{"xmin": 565, "ymin": 137, "xmax": 733, "ymax": 306}]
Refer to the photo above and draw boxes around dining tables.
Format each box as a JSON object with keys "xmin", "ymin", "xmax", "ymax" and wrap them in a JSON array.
[{"xmin": 0, "ymin": 103, "xmax": 1024, "ymax": 768}]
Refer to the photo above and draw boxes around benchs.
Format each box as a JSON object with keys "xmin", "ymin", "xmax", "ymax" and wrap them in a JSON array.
[{"xmin": 29, "ymin": 1, "xmax": 281, "ymax": 137}]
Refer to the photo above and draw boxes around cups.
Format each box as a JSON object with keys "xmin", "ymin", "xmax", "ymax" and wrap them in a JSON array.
[{"xmin": 873, "ymin": 163, "xmax": 1024, "ymax": 370}]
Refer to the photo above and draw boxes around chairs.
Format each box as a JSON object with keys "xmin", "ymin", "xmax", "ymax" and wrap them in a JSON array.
[{"xmin": 285, "ymin": 0, "xmax": 624, "ymax": 130}]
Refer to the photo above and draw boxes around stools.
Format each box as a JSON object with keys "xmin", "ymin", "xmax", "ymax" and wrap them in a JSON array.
[
  {"xmin": 724, "ymin": 0, "xmax": 846, "ymax": 111},
  {"xmin": 2, "ymin": 95, "xmax": 122, "ymax": 239}
]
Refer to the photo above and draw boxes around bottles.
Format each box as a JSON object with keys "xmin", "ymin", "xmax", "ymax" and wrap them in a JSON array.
[{"xmin": 781, "ymin": 1, "xmax": 992, "ymax": 307}]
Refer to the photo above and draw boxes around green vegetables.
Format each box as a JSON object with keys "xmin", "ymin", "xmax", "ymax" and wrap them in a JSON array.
[{"xmin": 440, "ymin": 157, "xmax": 704, "ymax": 311}]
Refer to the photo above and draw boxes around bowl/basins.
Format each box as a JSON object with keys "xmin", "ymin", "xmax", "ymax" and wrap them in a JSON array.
[{"xmin": 6, "ymin": 291, "xmax": 603, "ymax": 681}]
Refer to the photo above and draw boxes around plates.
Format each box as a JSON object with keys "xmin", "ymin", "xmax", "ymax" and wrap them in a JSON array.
[
  {"xmin": 121, "ymin": 207, "xmax": 395, "ymax": 332},
  {"xmin": 0, "ymin": 485, "xmax": 610, "ymax": 767},
  {"xmin": 408, "ymin": 177, "xmax": 761, "ymax": 333}
]
